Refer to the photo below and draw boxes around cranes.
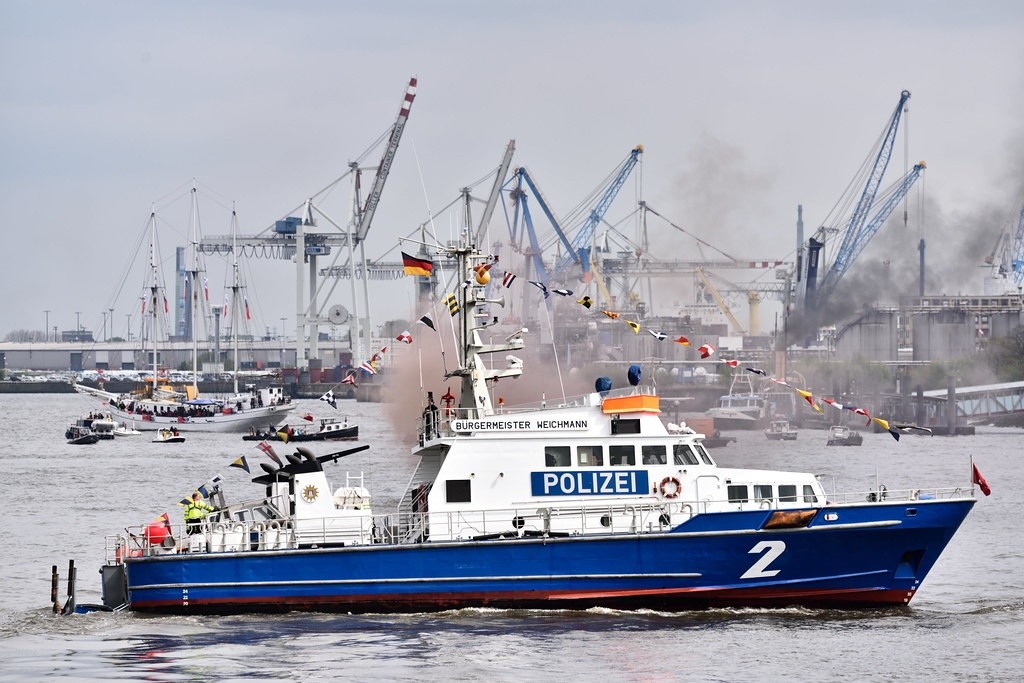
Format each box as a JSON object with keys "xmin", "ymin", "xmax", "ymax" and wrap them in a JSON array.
[{"xmin": 198, "ymin": 76, "xmax": 930, "ymax": 366}]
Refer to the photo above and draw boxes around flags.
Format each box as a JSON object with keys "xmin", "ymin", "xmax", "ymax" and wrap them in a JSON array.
[
  {"xmin": 401, "ymin": 252, "xmax": 432, "ymax": 278},
  {"xmin": 503, "ymin": 271, "xmax": 934, "ymax": 441},
  {"xmin": 142, "ymin": 260, "xmax": 493, "ymax": 545},
  {"xmin": 971, "ymin": 462, "xmax": 991, "ymax": 496}
]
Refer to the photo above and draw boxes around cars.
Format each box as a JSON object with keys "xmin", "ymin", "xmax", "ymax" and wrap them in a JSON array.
[{"xmin": 19, "ymin": 372, "xmax": 232, "ymax": 382}]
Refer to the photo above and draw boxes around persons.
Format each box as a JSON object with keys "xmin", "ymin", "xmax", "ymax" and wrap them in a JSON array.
[
  {"xmin": 423, "ymin": 398, "xmax": 440, "ymax": 439},
  {"xmin": 111, "ymin": 397, "xmax": 303, "ymax": 438},
  {"xmin": 184, "ymin": 492, "xmax": 216, "ymax": 535}
]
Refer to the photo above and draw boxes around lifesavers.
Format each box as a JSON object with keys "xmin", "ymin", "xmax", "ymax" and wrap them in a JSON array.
[
  {"xmin": 660, "ymin": 476, "xmax": 682, "ymax": 498},
  {"xmin": 776, "ymin": 425, "xmax": 782, "ymax": 432}
]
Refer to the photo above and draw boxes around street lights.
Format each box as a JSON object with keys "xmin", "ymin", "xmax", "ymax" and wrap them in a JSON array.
[
  {"xmin": 110, "ymin": 310, "xmax": 114, "ymax": 341},
  {"xmin": 74, "ymin": 311, "xmax": 81, "ymax": 340},
  {"xmin": 44, "ymin": 311, "xmax": 51, "ymax": 341},
  {"xmin": 280, "ymin": 317, "xmax": 286, "ymax": 339},
  {"xmin": 101, "ymin": 312, "xmax": 108, "ymax": 342},
  {"xmin": 126, "ymin": 313, "xmax": 133, "ymax": 341}
]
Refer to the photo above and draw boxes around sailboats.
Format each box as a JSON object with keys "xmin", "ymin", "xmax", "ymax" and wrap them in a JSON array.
[{"xmin": 70, "ymin": 176, "xmax": 299, "ymax": 434}]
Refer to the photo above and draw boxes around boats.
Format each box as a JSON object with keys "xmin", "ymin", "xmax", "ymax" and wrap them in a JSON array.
[
  {"xmin": 152, "ymin": 428, "xmax": 185, "ymax": 444},
  {"xmin": 667, "ymin": 418, "xmax": 735, "ymax": 448},
  {"xmin": 75, "ymin": 231, "xmax": 976, "ymax": 612},
  {"xmin": 242, "ymin": 417, "xmax": 359, "ymax": 441},
  {"xmin": 764, "ymin": 421, "xmax": 797, "ymax": 440},
  {"xmin": 826, "ymin": 426, "xmax": 865, "ymax": 447},
  {"xmin": 68, "ymin": 416, "xmax": 141, "ymax": 444},
  {"xmin": 703, "ymin": 368, "xmax": 777, "ymax": 430}
]
